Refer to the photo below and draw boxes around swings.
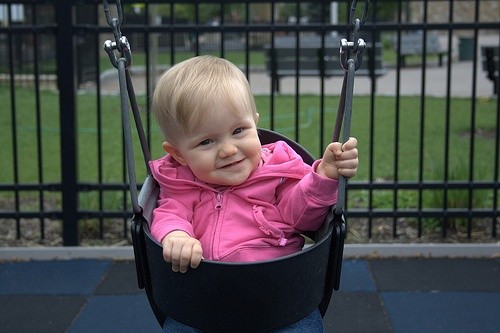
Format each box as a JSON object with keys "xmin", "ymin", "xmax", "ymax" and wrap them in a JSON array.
[{"xmin": 99, "ymin": 0, "xmax": 370, "ymax": 331}]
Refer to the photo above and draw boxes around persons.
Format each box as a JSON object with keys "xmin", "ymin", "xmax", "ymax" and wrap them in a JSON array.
[{"xmin": 148, "ymin": 55, "xmax": 359, "ymax": 333}]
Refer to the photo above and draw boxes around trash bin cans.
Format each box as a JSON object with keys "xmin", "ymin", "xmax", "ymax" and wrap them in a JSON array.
[{"xmin": 459, "ymin": 38, "xmax": 474, "ymax": 60}]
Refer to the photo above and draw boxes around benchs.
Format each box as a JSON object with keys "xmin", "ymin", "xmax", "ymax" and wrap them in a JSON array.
[
  {"xmin": 265, "ymin": 43, "xmax": 388, "ymax": 94},
  {"xmin": 481, "ymin": 45, "xmax": 500, "ymax": 98},
  {"xmin": 395, "ymin": 34, "xmax": 449, "ymax": 67}
]
